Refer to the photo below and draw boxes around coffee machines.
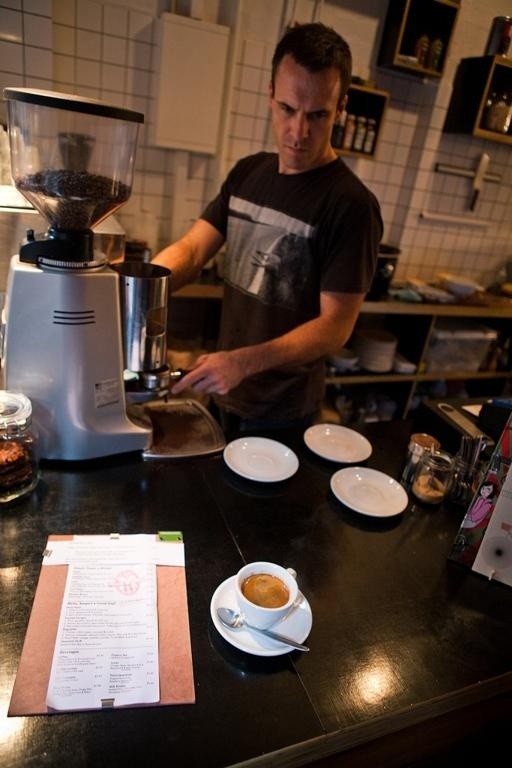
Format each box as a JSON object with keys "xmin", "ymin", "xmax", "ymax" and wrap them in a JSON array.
[{"xmin": 2, "ymin": 85, "xmax": 227, "ymax": 462}]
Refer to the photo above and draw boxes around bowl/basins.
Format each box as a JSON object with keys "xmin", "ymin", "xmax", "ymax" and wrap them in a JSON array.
[
  {"xmin": 438, "ymin": 271, "xmax": 485, "ymax": 295},
  {"xmin": 333, "ymin": 349, "xmax": 357, "ymax": 369}
]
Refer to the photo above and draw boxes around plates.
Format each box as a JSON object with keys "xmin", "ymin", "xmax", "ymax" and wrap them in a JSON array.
[
  {"xmin": 223, "ymin": 435, "xmax": 300, "ymax": 484},
  {"xmin": 356, "ymin": 335, "xmax": 398, "ymax": 372},
  {"xmin": 303, "ymin": 424, "xmax": 412, "ymax": 522},
  {"xmin": 208, "ymin": 574, "xmax": 314, "ymax": 660}
]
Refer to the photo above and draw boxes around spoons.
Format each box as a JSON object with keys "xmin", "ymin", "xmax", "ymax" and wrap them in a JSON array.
[{"xmin": 215, "ymin": 606, "xmax": 311, "ymax": 657}]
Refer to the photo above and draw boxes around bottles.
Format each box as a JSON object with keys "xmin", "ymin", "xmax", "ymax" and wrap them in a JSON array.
[
  {"xmin": 329, "ymin": 94, "xmax": 378, "ymax": 154},
  {"xmin": 0, "ymin": 389, "xmax": 41, "ymax": 502},
  {"xmin": 401, "ymin": 430, "xmax": 495, "ymax": 514},
  {"xmin": 479, "ymin": 91, "xmax": 511, "ymax": 138},
  {"xmin": 414, "ymin": 33, "xmax": 442, "ymax": 68}
]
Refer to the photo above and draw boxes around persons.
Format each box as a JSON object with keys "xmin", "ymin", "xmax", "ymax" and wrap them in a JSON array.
[{"xmin": 148, "ymin": 16, "xmax": 387, "ymax": 443}]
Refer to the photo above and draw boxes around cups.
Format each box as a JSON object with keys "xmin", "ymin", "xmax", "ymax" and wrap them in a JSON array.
[{"xmin": 237, "ymin": 561, "xmax": 298, "ymax": 630}]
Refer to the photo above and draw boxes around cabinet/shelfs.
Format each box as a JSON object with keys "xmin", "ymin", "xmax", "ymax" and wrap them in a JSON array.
[
  {"xmin": 330, "ymin": 1, "xmax": 512, "ymax": 162},
  {"xmin": 167, "ymin": 288, "xmax": 511, "ymax": 424}
]
